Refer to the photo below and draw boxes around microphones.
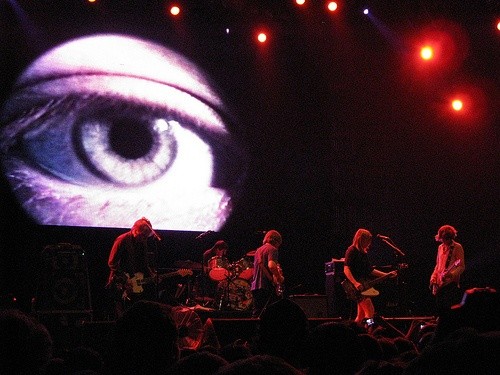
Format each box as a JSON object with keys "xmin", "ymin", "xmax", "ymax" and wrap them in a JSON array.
[
  {"xmin": 376, "ymin": 234, "xmax": 390, "ymax": 239},
  {"xmin": 196, "ymin": 231, "xmax": 211, "ymax": 239}
]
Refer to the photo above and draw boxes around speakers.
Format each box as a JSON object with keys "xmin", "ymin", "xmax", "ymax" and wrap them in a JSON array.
[
  {"xmin": 290, "ymin": 295, "xmax": 328, "ymax": 318},
  {"xmin": 326, "ymin": 273, "xmax": 355, "ymax": 317}
]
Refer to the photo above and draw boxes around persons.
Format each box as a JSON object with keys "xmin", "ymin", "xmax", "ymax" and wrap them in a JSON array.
[
  {"xmin": 108, "ymin": 219, "xmax": 152, "ymax": 314},
  {"xmin": 429, "ymin": 224, "xmax": 465, "ymax": 313},
  {"xmin": 201, "ymin": 241, "xmax": 228, "ymax": 288},
  {"xmin": 343, "ymin": 229, "xmax": 396, "ymax": 327},
  {"xmin": 251, "ymin": 230, "xmax": 284, "ymax": 308},
  {"xmin": 0, "ymin": 289, "xmax": 500, "ymax": 375}
]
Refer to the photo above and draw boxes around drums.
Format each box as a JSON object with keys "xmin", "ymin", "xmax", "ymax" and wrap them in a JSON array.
[
  {"xmin": 216, "ymin": 277, "xmax": 253, "ymax": 311},
  {"xmin": 207, "ymin": 256, "xmax": 230, "ymax": 281}
]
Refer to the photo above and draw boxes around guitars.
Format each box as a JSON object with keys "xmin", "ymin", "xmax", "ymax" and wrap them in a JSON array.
[
  {"xmin": 430, "ymin": 258, "xmax": 461, "ymax": 295},
  {"xmin": 340, "ymin": 263, "xmax": 409, "ymax": 305},
  {"xmin": 273, "ymin": 263, "xmax": 284, "ymax": 285},
  {"xmin": 110, "ymin": 267, "xmax": 193, "ymax": 294}
]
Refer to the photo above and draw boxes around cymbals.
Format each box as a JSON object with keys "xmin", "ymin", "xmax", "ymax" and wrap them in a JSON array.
[
  {"xmin": 247, "ymin": 249, "xmax": 257, "ymax": 257},
  {"xmin": 174, "ymin": 259, "xmax": 208, "ymax": 271}
]
